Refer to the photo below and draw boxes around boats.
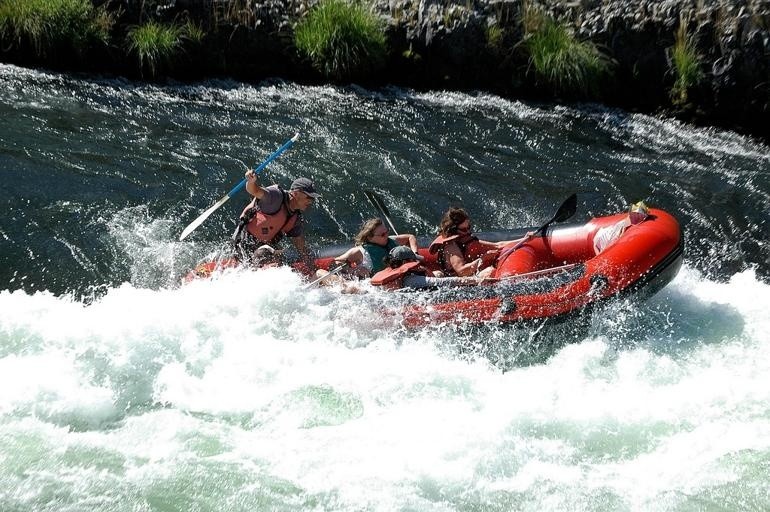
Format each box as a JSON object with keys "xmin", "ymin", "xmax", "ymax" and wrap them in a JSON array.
[{"xmin": 176, "ymin": 208, "xmax": 684, "ymax": 343}]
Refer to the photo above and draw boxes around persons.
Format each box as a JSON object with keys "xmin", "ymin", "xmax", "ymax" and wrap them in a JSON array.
[
  {"xmin": 252, "ymin": 244, "xmax": 363, "ymax": 300},
  {"xmin": 428, "ymin": 207, "xmax": 535, "ymax": 280},
  {"xmin": 388, "ymin": 245, "xmax": 485, "ymax": 296},
  {"xmin": 233, "ymin": 169, "xmax": 314, "ymax": 269},
  {"xmin": 336, "ymin": 218, "xmax": 444, "ymax": 287}
]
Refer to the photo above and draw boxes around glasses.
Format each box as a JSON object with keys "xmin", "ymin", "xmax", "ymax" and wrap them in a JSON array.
[{"xmin": 374, "ymin": 230, "xmax": 390, "ymax": 237}]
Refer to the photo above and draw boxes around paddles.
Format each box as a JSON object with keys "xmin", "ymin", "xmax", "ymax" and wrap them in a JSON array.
[
  {"xmin": 495, "ymin": 193, "xmax": 577, "ymax": 264},
  {"xmin": 364, "ymin": 188, "xmax": 400, "ymax": 236},
  {"xmin": 179, "ymin": 132, "xmax": 301, "ymax": 242}
]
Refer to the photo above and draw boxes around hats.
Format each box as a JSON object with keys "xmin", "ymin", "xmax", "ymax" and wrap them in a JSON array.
[
  {"xmin": 389, "ymin": 244, "xmax": 424, "ymax": 261},
  {"xmin": 290, "ymin": 177, "xmax": 323, "ymax": 198}
]
